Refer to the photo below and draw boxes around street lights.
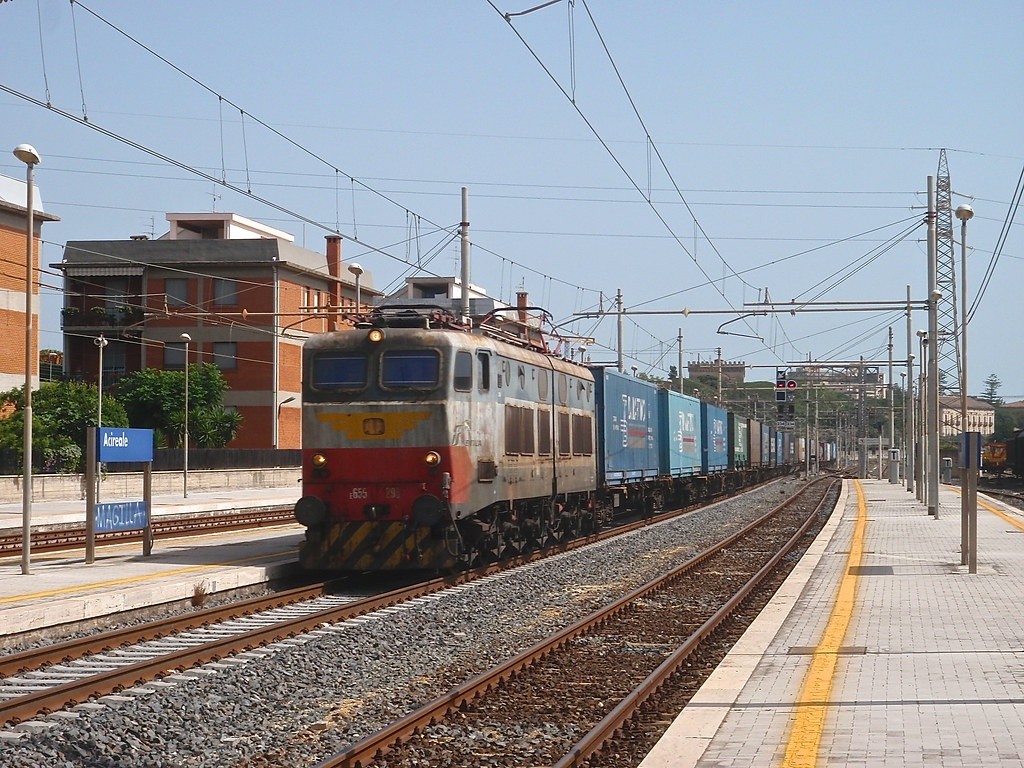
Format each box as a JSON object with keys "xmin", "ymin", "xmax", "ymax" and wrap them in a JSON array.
[
  {"xmin": 578, "ymin": 346, "xmax": 586, "ymax": 363},
  {"xmin": 956, "ymin": 204, "xmax": 975, "ymax": 565},
  {"xmin": 180, "ymin": 333, "xmax": 192, "ymax": 498},
  {"xmin": 13, "ymin": 144, "xmax": 41, "ymax": 575},
  {"xmin": 668, "ymin": 380, "xmax": 672, "ymax": 391},
  {"xmin": 94, "ymin": 333, "xmax": 108, "ymax": 504},
  {"xmin": 348, "ymin": 263, "xmax": 364, "ymax": 329},
  {"xmin": 900, "ymin": 372, "xmax": 907, "ymax": 487},
  {"xmin": 631, "ymin": 365, "xmax": 638, "ymax": 377},
  {"xmin": 916, "ymin": 330, "xmax": 928, "ymax": 500}
]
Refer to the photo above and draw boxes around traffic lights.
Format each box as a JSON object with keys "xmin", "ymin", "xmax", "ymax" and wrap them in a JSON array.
[
  {"xmin": 786, "ymin": 379, "xmax": 797, "ymax": 391},
  {"xmin": 775, "ymin": 380, "xmax": 786, "ymax": 402}
]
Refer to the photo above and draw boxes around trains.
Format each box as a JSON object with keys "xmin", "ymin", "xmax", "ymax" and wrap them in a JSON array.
[{"xmin": 294, "ymin": 305, "xmax": 836, "ymax": 571}]
